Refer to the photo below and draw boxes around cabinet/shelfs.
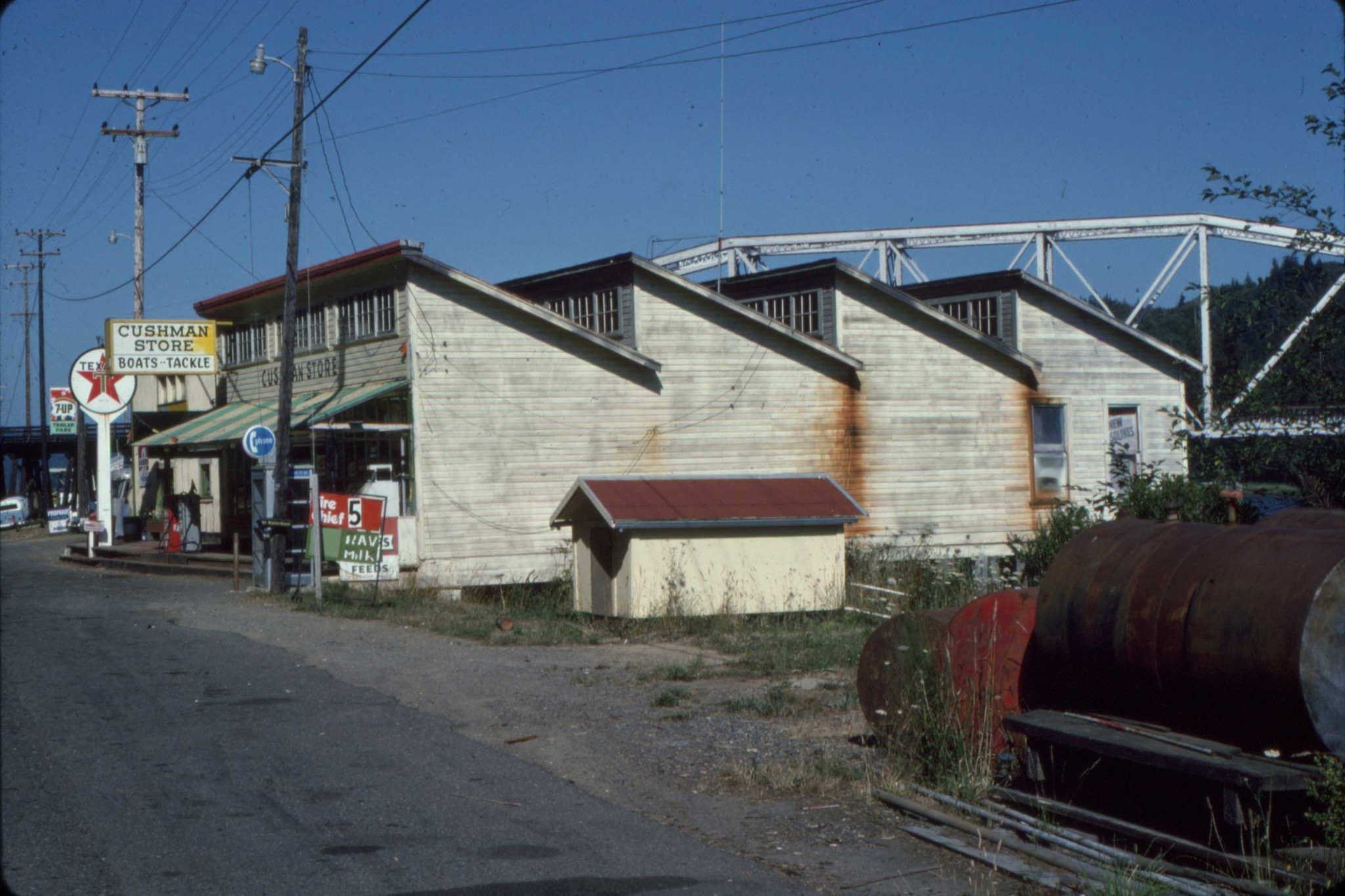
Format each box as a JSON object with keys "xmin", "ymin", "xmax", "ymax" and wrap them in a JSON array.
[{"xmin": 250, "ymin": 464, "xmax": 314, "ymax": 589}]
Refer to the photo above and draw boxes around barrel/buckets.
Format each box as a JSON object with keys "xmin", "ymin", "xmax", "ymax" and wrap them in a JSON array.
[
  {"xmin": 856, "ymin": 587, "xmax": 1038, "ymax": 779},
  {"xmin": 1034, "ymin": 504, "xmax": 1345, "ymax": 770}
]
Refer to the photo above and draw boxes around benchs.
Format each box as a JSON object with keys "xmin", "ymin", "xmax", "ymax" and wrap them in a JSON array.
[{"xmin": 1002, "ymin": 710, "xmax": 1320, "ymax": 855}]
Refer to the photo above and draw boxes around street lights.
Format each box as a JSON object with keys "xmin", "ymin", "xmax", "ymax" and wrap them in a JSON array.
[
  {"xmin": 246, "ymin": 41, "xmax": 308, "ymax": 599},
  {"xmin": 108, "ymin": 226, "xmax": 144, "ymax": 319}
]
